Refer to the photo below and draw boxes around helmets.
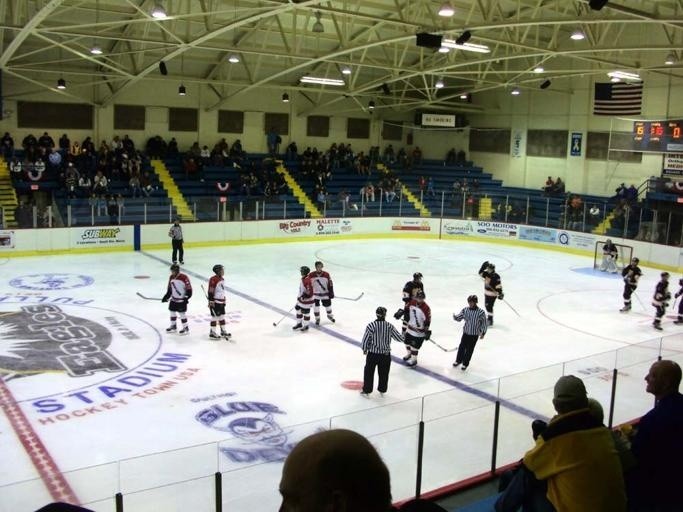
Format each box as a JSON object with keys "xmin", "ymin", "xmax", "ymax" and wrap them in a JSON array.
[
  {"xmin": 606, "ymin": 239, "xmax": 669, "ymax": 277},
  {"xmin": 376, "ymin": 263, "xmax": 495, "ymax": 318},
  {"xmin": 171, "ymin": 262, "xmax": 323, "ymax": 275}
]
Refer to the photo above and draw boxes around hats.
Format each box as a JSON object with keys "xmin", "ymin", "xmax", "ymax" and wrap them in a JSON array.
[{"xmin": 554, "ymin": 376, "xmax": 588, "ymax": 402}]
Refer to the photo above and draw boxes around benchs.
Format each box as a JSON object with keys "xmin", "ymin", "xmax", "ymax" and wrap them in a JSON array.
[{"xmin": 448, "ymin": 491, "xmax": 507, "ymax": 512}]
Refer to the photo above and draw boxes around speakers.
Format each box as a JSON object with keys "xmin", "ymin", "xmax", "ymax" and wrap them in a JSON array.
[
  {"xmin": 540, "ymin": 80, "xmax": 550, "ymax": 89},
  {"xmin": 160, "ymin": 62, "xmax": 167, "ymax": 75},
  {"xmin": 590, "ymin": 0, "xmax": 608, "ymax": 10},
  {"xmin": 416, "ymin": 33, "xmax": 442, "ymax": 48},
  {"xmin": 382, "ymin": 84, "xmax": 390, "ymax": 95},
  {"xmin": 456, "ymin": 32, "xmax": 471, "ymax": 45}
]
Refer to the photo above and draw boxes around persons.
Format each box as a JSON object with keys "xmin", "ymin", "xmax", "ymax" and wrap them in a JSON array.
[
  {"xmin": 478, "ymin": 262, "xmax": 502, "ymax": 325},
  {"xmin": 615, "ymin": 182, "xmax": 637, "ymax": 203},
  {"xmin": 308, "ymin": 260, "xmax": 335, "ymax": 323},
  {"xmin": 619, "ymin": 255, "xmax": 682, "ymax": 330},
  {"xmin": 504, "ymin": 357, "xmax": 682, "ymax": 511},
  {"xmin": 498, "ymin": 195, "xmax": 534, "ymax": 226},
  {"xmin": 361, "ymin": 306, "xmax": 407, "ymax": 395},
  {"xmin": 292, "ymin": 266, "xmax": 312, "ymax": 332},
  {"xmin": 161, "ymin": 264, "xmax": 191, "ymax": 334},
  {"xmin": 402, "ymin": 271, "xmax": 425, "ymax": 338},
  {"xmin": 393, "ymin": 292, "xmax": 430, "ymax": 367},
  {"xmin": 453, "ymin": 295, "xmax": 486, "ymax": 371},
  {"xmin": 168, "ymin": 219, "xmax": 185, "ymax": 266},
  {"xmin": 277, "ymin": 428, "xmax": 450, "ymax": 511},
  {"xmin": 543, "ymin": 175, "xmax": 565, "ymax": 198},
  {"xmin": 1, "ymin": 127, "xmax": 480, "ymax": 228},
  {"xmin": 599, "ymin": 239, "xmax": 618, "ymax": 274},
  {"xmin": 204, "ymin": 264, "xmax": 231, "ymax": 340},
  {"xmin": 560, "ymin": 191, "xmax": 601, "ymax": 231}
]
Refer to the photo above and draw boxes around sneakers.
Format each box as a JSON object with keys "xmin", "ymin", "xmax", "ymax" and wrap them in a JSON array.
[
  {"xmin": 293, "ymin": 317, "xmax": 335, "ymax": 332},
  {"xmin": 166, "ymin": 325, "xmax": 231, "ymax": 339},
  {"xmin": 360, "ymin": 354, "xmax": 467, "ymax": 396},
  {"xmin": 620, "ymin": 305, "xmax": 663, "ymax": 330}
]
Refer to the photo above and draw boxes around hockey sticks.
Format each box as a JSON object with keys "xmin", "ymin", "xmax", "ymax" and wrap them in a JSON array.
[
  {"xmin": 485, "ymin": 279, "xmax": 520, "ymax": 319},
  {"xmin": 273, "ymin": 300, "xmax": 299, "ymax": 326},
  {"xmin": 334, "ymin": 292, "xmax": 364, "ymax": 301},
  {"xmin": 399, "ymin": 317, "xmax": 458, "ymax": 352},
  {"xmin": 201, "ymin": 284, "xmax": 228, "ymax": 341},
  {"xmin": 137, "ymin": 292, "xmax": 163, "ymax": 300}
]
